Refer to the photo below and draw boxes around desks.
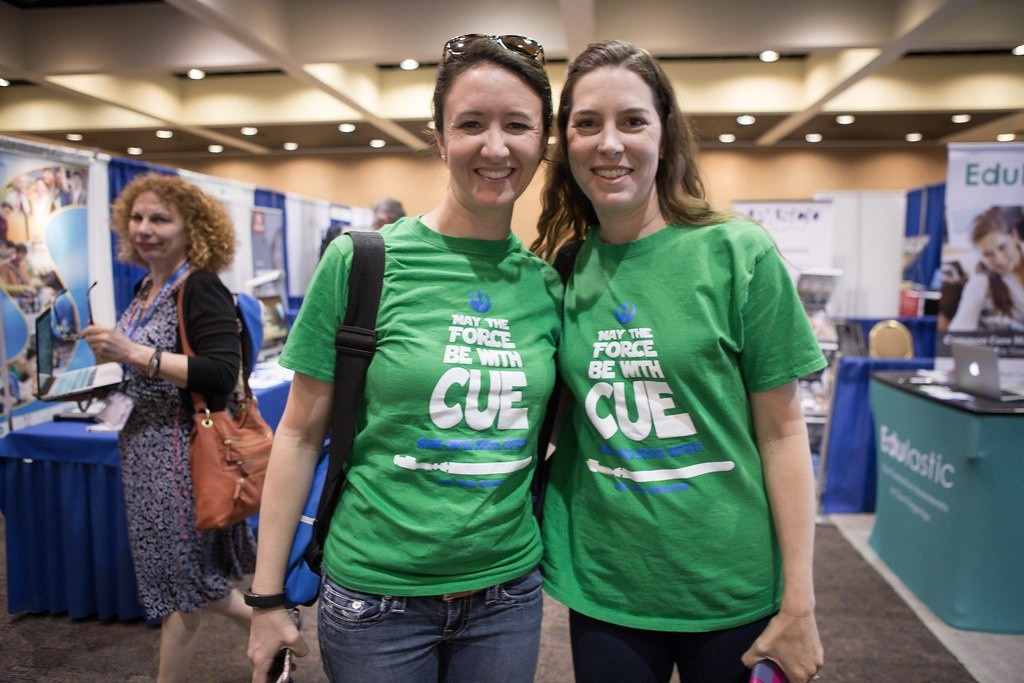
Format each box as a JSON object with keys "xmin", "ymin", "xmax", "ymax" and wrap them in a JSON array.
[
  {"xmin": 866, "ymin": 369, "xmax": 1024, "ymax": 635},
  {"xmin": 0, "ymin": 353, "xmax": 293, "ymax": 628}
]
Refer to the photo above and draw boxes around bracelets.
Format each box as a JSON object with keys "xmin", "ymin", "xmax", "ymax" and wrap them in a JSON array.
[
  {"xmin": 244, "ymin": 585, "xmax": 293, "ymax": 608},
  {"xmin": 148, "ymin": 352, "xmax": 161, "ymax": 380}
]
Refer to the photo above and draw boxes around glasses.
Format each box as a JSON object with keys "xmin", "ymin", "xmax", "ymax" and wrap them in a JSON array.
[
  {"xmin": 51, "ymin": 279, "xmax": 106, "ymax": 344},
  {"xmin": 439, "ymin": 33, "xmax": 547, "ymax": 70}
]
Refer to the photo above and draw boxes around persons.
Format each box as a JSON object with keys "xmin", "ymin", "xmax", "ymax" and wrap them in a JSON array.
[
  {"xmin": 947, "ymin": 206, "xmax": 1024, "ymax": 344},
  {"xmin": 79, "ymin": 174, "xmax": 250, "ymax": 683},
  {"xmin": 243, "ymin": 37, "xmax": 565, "ymax": 683},
  {"xmin": 1, "ymin": 164, "xmax": 89, "ymax": 419},
  {"xmin": 373, "ymin": 200, "xmax": 405, "ymax": 231},
  {"xmin": 533, "ymin": 40, "xmax": 827, "ymax": 683}
]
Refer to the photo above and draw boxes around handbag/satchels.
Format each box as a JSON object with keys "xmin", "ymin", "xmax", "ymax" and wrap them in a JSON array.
[{"xmin": 188, "ymin": 383, "xmax": 276, "ymax": 532}]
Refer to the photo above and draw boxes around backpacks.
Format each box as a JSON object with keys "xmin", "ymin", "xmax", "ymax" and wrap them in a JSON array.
[{"xmin": 276, "ymin": 228, "xmax": 385, "ymax": 607}]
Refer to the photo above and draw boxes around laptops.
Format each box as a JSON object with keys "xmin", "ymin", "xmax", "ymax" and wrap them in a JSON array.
[
  {"xmin": 35, "ymin": 307, "xmax": 125, "ymax": 401},
  {"xmin": 952, "ymin": 342, "xmax": 1024, "ymax": 404}
]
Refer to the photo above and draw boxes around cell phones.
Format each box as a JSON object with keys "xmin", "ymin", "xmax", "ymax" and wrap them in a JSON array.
[
  {"xmin": 271, "ymin": 608, "xmax": 300, "ymax": 683},
  {"xmin": 749, "ymin": 659, "xmax": 790, "ymax": 683}
]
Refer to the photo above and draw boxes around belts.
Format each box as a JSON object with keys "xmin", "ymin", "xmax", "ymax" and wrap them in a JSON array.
[{"xmin": 404, "ymin": 567, "xmax": 535, "ymax": 602}]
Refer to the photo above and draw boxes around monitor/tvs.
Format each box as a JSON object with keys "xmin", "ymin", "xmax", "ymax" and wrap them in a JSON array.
[
  {"xmin": 795, "ymin": 275, "xmax": 842, "ymax": 319},
  {"xmin": 258, "ymin": 294, "xmax": 288, "ymax": 343}
]
